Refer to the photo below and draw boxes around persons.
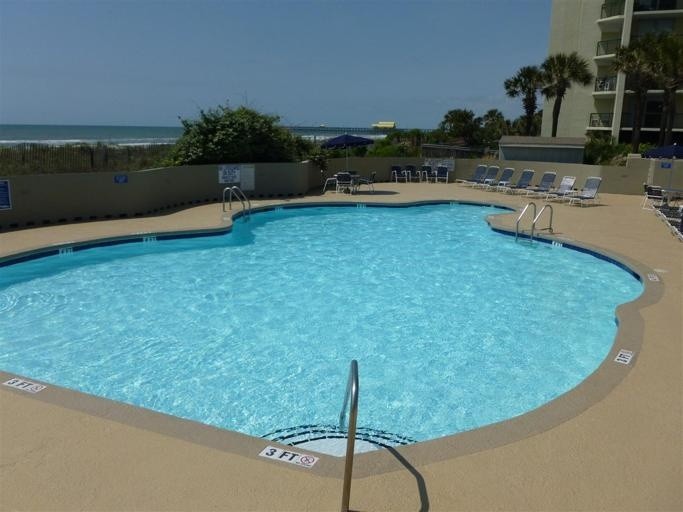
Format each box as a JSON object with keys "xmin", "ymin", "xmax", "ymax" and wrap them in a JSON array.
[{"xmin": 641, "ymin": 142, "xmax": 683, "ymax": 190}]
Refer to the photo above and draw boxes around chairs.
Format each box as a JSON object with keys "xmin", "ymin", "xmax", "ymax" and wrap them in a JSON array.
[
  {"xmin": 454, "ymin": 163, "xmax": 604, "ymax": 210},
  {"xmin": 389, "ymin": 164, "xmax": 449, "ymax": 185},
  {"xmin": 639, "ymin": 181, "xmax": 683, "ymax": 243},
  {"xmin": 324, "ymin": 168, "xmax": 377, "ymax": 195}
]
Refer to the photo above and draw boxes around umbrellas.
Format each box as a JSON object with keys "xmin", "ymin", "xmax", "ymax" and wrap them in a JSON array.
[{"xmin": 321, "ymin": 132, "xmax": 374, "ymax": 172}]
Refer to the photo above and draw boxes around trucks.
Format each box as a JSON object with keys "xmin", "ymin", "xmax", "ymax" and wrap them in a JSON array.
[{"xmin": 369, "ymin": 120, "xmax": 397, "ymax": 128}]
